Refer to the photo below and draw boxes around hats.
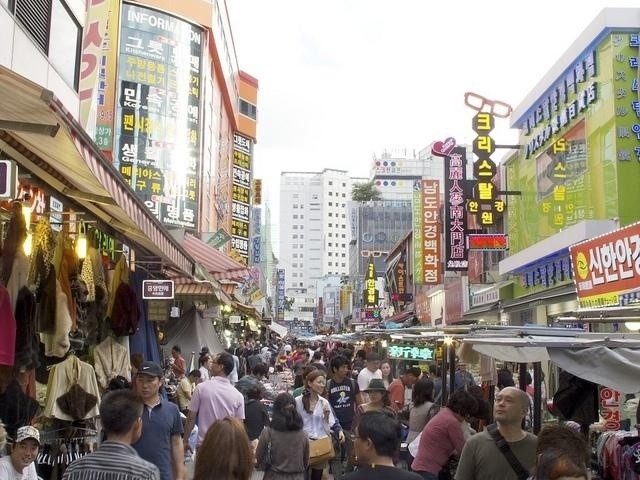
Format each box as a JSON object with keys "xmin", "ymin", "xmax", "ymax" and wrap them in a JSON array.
[
  {"xmin": 363, "ymin": 379, "xmax": 386, "ymax": 393},
  {"xmin": 138, "ymin": 361, "xmax": 160, "ymax": 377},
  {"xmin": 15, "ymin": 425, "xmax": 41, "ymax": 445}
]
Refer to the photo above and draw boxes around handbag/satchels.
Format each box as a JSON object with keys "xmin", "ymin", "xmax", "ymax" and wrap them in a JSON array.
[
  {"xmin": 255, "ymin": 441, "xmax": 272, "ymax": 471},
  {"xmin": 309, "ymin": 434, "xmax": 335, "ymax": 465}
]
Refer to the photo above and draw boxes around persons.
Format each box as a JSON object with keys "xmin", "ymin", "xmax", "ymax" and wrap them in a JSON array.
[{"xmin": 0, "ymin": 331, "xmax": 640, "ymax": 480}]
